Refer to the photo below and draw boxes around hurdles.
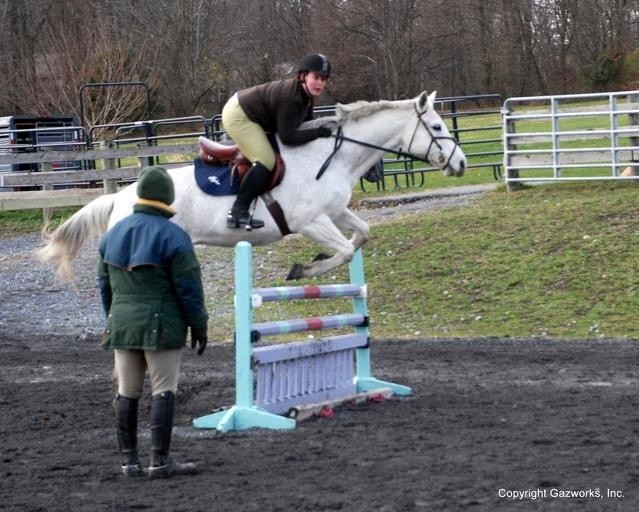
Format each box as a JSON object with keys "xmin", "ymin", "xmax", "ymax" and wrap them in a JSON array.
[{"xmin": 192, "ymin": 242, "xmax": 412, "ymax": 432}]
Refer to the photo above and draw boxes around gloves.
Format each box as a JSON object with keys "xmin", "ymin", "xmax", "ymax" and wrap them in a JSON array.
[
  {"xmin": 318, "ymin": 126, "xmax": 332, "ymax": 138},
  {"xmin": 191, "ymin": 327, "xmax": 208, "ymax": 355}
]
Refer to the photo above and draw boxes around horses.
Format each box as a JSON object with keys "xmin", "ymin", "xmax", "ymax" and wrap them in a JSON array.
[{"xmin": 39, "ymin": 88, "xmax": 470, "ymax": 297}]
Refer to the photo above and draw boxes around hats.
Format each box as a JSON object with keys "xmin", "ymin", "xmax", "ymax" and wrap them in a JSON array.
[{"xmin": 135, "ymin": 167, "xmax": 174, "ymax": 205}]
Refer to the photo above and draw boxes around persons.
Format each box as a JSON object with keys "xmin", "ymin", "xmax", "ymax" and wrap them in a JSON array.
[
  {"xmin": 95, "ymin": 163, "xmax": 210, "ymax": 482},
  {"xmin": 222, "ymin": 51, "xmax": 334, "ymax": 230}
]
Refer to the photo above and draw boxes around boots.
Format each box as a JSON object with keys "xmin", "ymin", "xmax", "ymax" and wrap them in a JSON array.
[
  {"xmin": 113, "ymin": 393, "xmax": 140, "ymax": 476},
  {"xmin": 226, "ymin": 162, "xmax": 271, "ymax": 229},
  {"xmin": 147, "ymin": 390, "xmax": 197, "ymax": 480}
]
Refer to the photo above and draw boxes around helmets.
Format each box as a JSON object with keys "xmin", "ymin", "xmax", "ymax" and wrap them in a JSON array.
[{"xmin": 298, "ymin": 52, "xmax": 331, "ymax": 79}]
